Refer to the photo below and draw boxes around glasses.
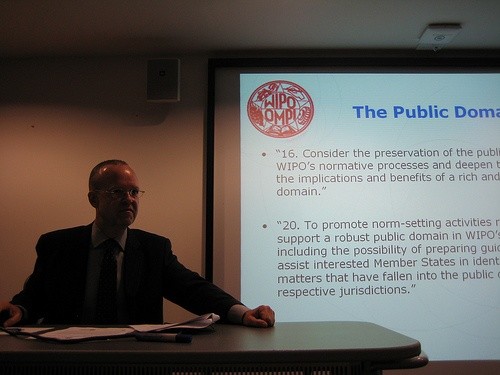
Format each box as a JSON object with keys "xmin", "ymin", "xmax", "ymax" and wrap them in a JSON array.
[{"xmin": 95, "ymin": 186, "xmax": 145, "ymax": 201}]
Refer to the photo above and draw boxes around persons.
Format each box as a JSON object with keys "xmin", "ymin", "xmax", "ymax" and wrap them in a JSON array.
[{"xmin": 0, "ymin": 159, "xmax": 276, "ymax": 329}]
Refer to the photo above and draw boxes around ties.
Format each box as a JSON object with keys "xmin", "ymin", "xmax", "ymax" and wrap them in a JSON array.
[{"xmin": 95, "ymin": 240, "xmax": 120, "ymax": 324}]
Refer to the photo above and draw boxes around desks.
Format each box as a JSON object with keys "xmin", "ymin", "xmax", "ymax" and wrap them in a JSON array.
[{"xmin": 0, "ymin": 321, "xmax": 428, "ymax": 375}]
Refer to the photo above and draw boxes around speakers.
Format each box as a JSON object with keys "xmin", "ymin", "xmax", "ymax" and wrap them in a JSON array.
[{"xmin": 147, "ymin": 57, "xmax": 181, "ymax": 103}]
